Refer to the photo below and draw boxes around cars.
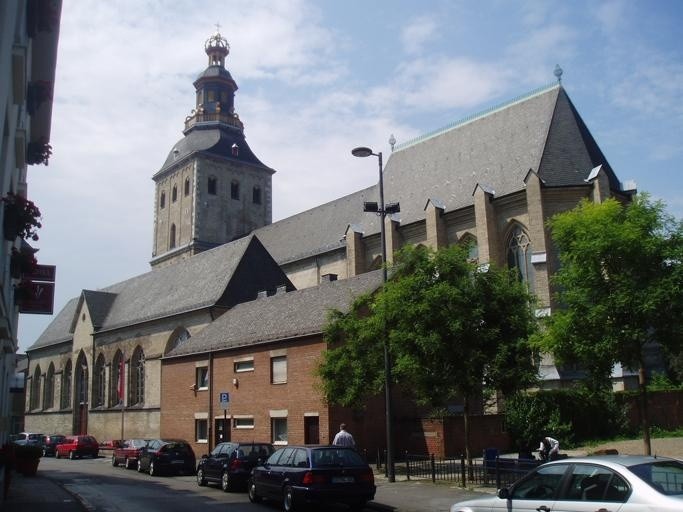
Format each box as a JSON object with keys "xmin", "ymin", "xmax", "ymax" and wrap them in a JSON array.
[
  {"xmin": 451, "ymin": 454, "xmax": 683, "ymax": 512},
  {"xmin": 110, "ymin": 436, "xmax": 377, "ymax": 511}
]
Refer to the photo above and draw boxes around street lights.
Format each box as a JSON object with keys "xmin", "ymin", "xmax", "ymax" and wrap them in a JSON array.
[{"xmin": 349, "ymin": 146, "xmax": 397, "ymax": 482}]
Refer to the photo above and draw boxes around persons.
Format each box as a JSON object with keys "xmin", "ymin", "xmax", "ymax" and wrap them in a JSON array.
[
  {"xmin": 331, "ymin": 423, "xmax": 356, "ymax": 465},
  {"xmin": 534, "ymin": 436, "xmax": 560, "ymax": 463}
]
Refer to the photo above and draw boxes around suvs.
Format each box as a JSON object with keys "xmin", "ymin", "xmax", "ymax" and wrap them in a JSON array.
[{"xmin": 9, "ymin": 431, "xmax": 101, "ymax": 461}]
